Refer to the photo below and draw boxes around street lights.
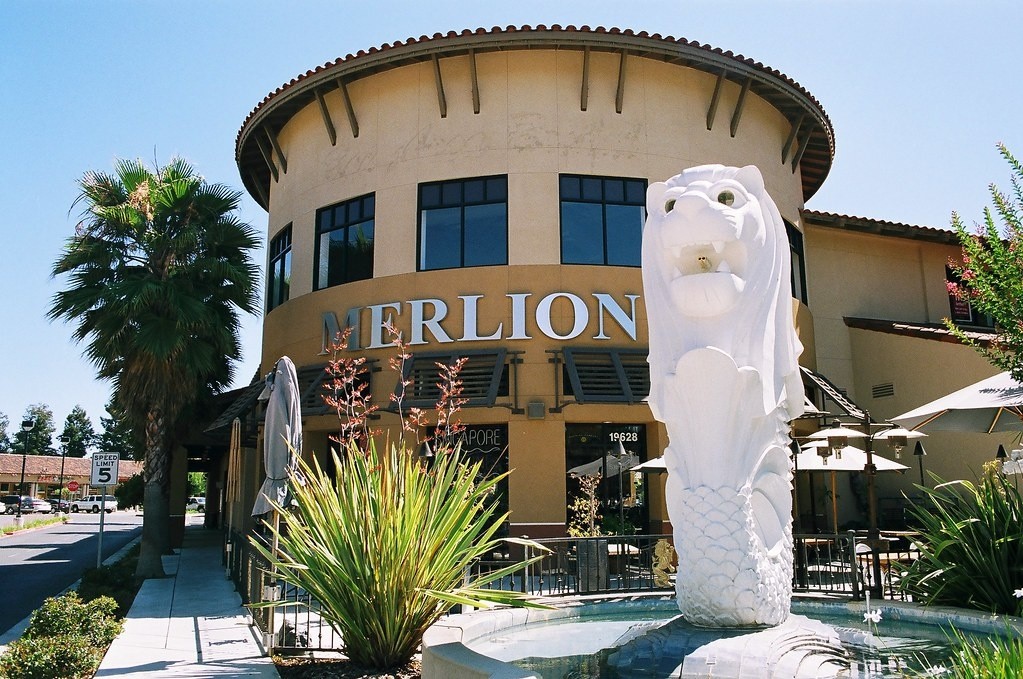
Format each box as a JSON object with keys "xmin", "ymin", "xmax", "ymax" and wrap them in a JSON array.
[
  {"xmin": 830, "ymin": 410, "xmax": 908, "ymax": 597},
  {"xmin": 56, "ymin": 437, "xmax": 71, "ymax": 512},
  {"xmin": 13, "ymin": 421, "xmax": 34, "ymax": 518}
]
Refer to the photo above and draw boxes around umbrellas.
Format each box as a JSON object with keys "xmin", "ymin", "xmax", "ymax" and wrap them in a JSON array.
[
  {"xmin": 629, "ymin": 363, "xmax": 1023, "ymax": 534},
  {"xmin": 250, "ymin": 355, "xmax": 303, "ymax": 587},
  {"xmin": 568, "ymin": 453, "xmax": 639, "ymax": 479},
  {"xmin": 567, "ymin": 455, "xmax": 616, "ymax": 473}
]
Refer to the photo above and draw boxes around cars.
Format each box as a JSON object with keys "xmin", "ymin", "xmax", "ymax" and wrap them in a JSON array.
[
  {"xmin": 46, "ymin": 499, "xmax": 69, "ymax": 514},
  {"xmin": 22, "ymin": 497, "xmax": 51, "ymax": 515}
]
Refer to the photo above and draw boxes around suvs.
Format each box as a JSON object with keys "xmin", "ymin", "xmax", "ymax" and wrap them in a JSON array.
[{"xmin": 0, "ymin": 495, "xmax": 34, "ymax": 516}]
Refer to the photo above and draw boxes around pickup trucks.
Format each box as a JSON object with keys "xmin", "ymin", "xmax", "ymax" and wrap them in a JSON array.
[{"xmin": 71, "ymin": 495, "xmax": 118, "ymax": 513}]
